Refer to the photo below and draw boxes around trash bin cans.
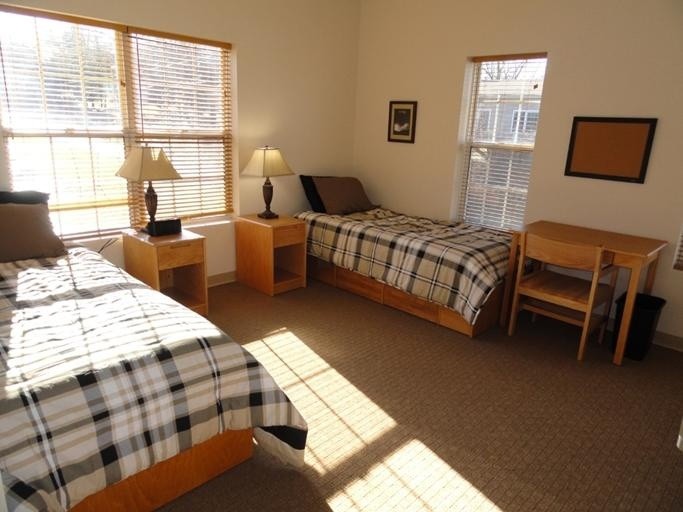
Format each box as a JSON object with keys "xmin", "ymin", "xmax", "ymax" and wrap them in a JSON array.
[{"xmin": 609, "ymin": 292, "xmax": 667, "ymax": 361}]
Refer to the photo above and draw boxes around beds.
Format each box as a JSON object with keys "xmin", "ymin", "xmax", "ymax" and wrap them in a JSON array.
[
  {"xmin": 1, "ymin": 241, "xmax": 310, "ymax": 511},
  {"xmin": 293, "ymin": 204, "xmax": 542, "ymax": 340}
]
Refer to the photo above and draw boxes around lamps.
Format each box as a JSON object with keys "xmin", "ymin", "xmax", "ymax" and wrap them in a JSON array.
[
  {"xmin": 115, "ymin": 144, "xmax": 183, "ymax": 234},
  {"xmin": 241, "ymin": 142, "xmax": 297, "ymax": 219}
]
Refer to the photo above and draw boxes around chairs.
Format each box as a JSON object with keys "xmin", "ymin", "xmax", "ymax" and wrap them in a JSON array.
[{"xmin": 507, "ymin": 230, "xmax": 619, "ymax": 362}]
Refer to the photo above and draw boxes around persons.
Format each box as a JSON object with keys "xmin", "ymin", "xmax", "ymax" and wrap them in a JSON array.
[{"xmin": 393, "ymin": 110, "xmax": 409, "ymax": 132}]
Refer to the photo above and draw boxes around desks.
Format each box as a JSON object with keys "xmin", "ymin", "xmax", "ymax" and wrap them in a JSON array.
[{"xmin": 496, "ymin": 220, "xmax": 669, "ymax": 366}]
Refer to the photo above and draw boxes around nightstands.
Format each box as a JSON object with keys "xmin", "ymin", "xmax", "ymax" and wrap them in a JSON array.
[
  {"xmin": 234, "ymin": 211, "xmax": 309, "ymax": 297},
  {"xmin": 123, "ymin": 228, "xmax": 210, "ymax": 318}
]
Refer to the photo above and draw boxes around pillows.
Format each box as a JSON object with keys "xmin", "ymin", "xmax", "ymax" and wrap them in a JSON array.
[
  {"xmin": 298, "ymin": 173, "xmax": 338, "ymax": 213},
  {"xmin": 1, "ymin": 190, "xmax": 50, "ymax": 203},
  {"xmin": 1, "ymin": 204, "xmax": 71, "ymax": 264},
  {"xmin": 311, "ymin": 175, "xmax": 381, "ymax": 216}
]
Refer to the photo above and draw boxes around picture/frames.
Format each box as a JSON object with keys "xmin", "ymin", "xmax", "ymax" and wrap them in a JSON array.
[
  {"xmin": 387, "ymin": 101, "xmax": 417, "ymax": 144},
  {"xmin": 564, "ymin": 116, "xmax": 659, "ymax": 185}
]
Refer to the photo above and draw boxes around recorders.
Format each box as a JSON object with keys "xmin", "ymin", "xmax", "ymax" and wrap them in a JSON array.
[{"xmin": 148, "ymin": 218, "xmax": 181, "ymax": 236}]
